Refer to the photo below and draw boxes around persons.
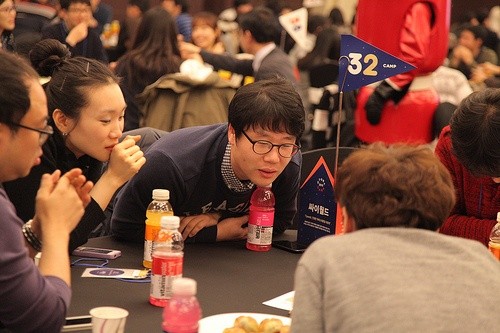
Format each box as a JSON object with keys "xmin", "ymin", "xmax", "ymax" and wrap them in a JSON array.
[
  {"xmin": 433, "ymin": 88, "xmax": 500, "ymax": 248},
  {"xmin": 91, "ymin": 77, "xmax": 304, "ymax": 247},
  {"xmin": 289, "ymin": 141, "xmax": 500, "ymax": 333},
  {"xmin": 0, "ymin": 0, "xmax": 500, "ymax": 139},
  {"xmin": 3, "ymin": 39, "xmax": 146, "ymax": 256},
  {"xmin": 0, "ymin": 48, "xmax": 94, "ymax": 333}
]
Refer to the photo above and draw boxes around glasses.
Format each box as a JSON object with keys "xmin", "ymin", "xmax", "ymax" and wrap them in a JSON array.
[
  {"xmin": 0, "ymin": 6, "xmax": 17, "ymax": 14},
  {"xmin": 7, "ymin": 117, "xmax": 54, "ymax": 145},
  {"xmin": 241, "ymin": 128, "xmax": 302, "ymax": 159}
]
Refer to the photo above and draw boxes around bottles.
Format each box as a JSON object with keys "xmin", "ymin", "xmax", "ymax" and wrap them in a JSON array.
[
  {"xmin": 143, "ymin": 189, "xmax": 174, "ymax": 268},
  {"xmin": 246, "ymin": 183, "xmax": 275, "ymax": 251},
  {"xmin": 160, "ymin": 277, "xmax": 202, "ymax": 333},
  {"xmin": 150, "ymin": 216, "xmax": 184, "ymax": 307}
]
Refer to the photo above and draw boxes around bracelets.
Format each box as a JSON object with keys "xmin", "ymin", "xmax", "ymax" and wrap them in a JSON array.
[{"xmin": 22, "ymin": 219, "xmax": 41, "ymax": 251}]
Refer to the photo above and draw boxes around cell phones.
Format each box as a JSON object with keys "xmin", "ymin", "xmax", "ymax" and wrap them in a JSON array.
[
  {"xmin": 62, "ymin": 315, "xmax": 92, "ymax": 328},
  {"xmin": 73, "ymin": 246, "xmax": 122, "ymax": 260},
  {"xmin": 273, "ymin": 240, "xmax": 308, "ymax": 252}
]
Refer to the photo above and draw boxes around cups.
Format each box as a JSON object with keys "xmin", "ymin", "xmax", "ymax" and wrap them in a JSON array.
[{"xmin": 89, "ymin": 307, "xmax": 129, "ymax": 333}]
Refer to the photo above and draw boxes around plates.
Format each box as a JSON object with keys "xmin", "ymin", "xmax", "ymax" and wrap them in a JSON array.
[{"xmin": 198, "ymin": 312, "xmax": 292, "ymax": 333}]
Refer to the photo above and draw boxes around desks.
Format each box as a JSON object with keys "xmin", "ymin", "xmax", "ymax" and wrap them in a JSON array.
[{"xmin": 60, "ymin": 230, "xmax": 303, "ymax": 333}]
